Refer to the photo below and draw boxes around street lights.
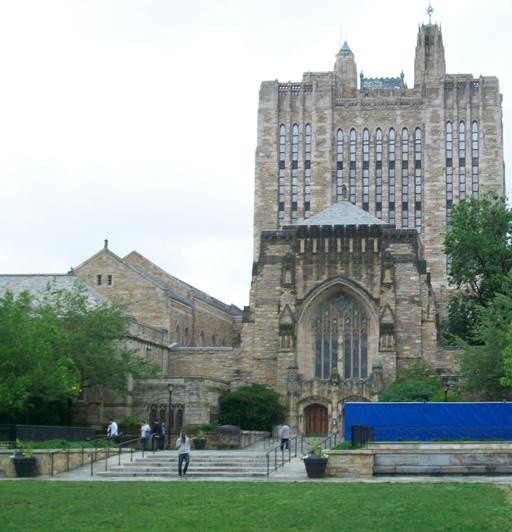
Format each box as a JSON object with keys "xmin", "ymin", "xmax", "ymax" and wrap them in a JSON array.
[
  {"xmin": 442, "ymin": 384, "xmax": 449, "ymax": 401},
  {"xmin": 165, "ymin": 383, "xmax": 176, "ymax": 450}
]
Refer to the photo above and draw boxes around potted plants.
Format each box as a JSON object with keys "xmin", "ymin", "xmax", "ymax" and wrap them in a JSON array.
[
  {"xmin": 10, "ymin": 442, "xmax": 38, "ymax": 476},
  {"xmin": 190, "ymin": 428, "xmax": 210, "ymax": 450},
  {"xmin": 302, "ymin": 434, "xmax": 327, "ymax": 479}
]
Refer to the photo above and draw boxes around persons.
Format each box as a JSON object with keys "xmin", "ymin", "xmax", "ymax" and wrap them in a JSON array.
[
  {"xmin": 176, "ymin": 431, "xmax": 191, "ymax": 476},
  {"xmin": 279, "ymin": 423, "xmax": 290, "ymax": 450},
  {"xmin": 107, "ymin": 418, "xmax": 166, "ymax": 450}
]
